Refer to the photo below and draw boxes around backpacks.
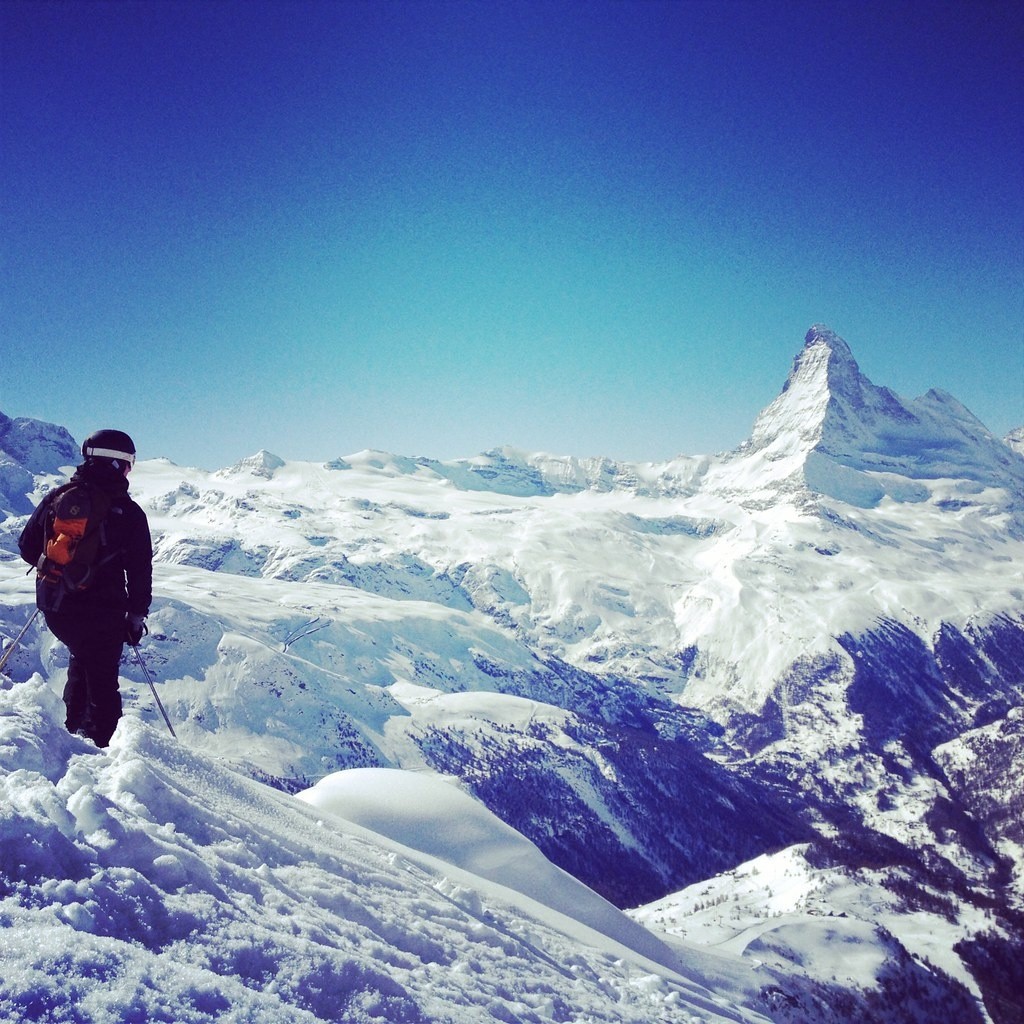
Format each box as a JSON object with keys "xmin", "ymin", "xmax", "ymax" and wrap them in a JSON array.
[{"xmin": 31, "ymin": 483, "xmax": 134, "ymax": 613}]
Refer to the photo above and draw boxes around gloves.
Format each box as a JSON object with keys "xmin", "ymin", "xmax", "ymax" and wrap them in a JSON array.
[{"xmin": 122, "ymin": 614, "xmax": 149, "ymax": 647}]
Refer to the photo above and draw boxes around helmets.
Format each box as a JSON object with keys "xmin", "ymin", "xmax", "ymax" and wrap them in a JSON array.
[{"xmin": 80, "ymin": 429, "xmax": 138, "ymax": 469}]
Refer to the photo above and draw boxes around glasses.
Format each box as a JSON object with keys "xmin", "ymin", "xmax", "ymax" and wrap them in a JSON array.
[{"xmin": 130, "ymin": 454, "xmax": 136, "ymax": 469}]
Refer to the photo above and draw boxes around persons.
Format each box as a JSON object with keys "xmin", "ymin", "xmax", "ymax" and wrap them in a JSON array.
[{"xmin": 19, "ymin": 431, "xmax": 153, "ymax": 748}]
